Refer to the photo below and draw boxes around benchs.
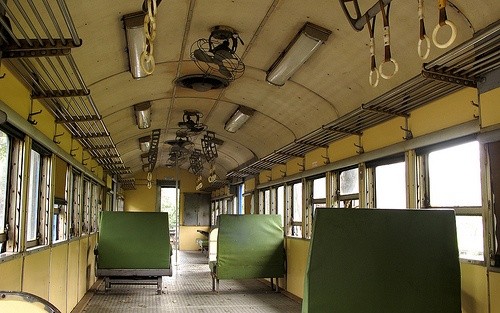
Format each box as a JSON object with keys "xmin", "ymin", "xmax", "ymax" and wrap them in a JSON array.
[{"xmin": 196, "ymin": 229, "xmax": 209, "ymax": 249}]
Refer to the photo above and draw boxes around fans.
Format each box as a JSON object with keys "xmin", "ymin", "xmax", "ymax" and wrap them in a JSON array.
[
  {"xmin": 174, "ymin": 110, "xmax": 209, "ymax": 137},
  {"xmin": 190, "ymin": 24, "xmax": 247, "ymax": 82}
]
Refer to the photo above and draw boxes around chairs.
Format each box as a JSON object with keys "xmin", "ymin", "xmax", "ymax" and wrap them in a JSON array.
[
  {"xmin": 206, "ymin": 213, "xmax": 287, "ymax": 292},
  {"xmin": 93, "ymin": 210, "xmax": 174, "ymax": 296},
  {"xmin": 302, "ymin": 205, "xmax": 463, "ymax": 312}
]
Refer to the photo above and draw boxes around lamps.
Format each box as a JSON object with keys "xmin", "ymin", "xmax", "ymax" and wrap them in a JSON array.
[
  {"xmin": 138, "ymin": 134, "xmax": 153, "ymax": 153},
  {"xmin": 263, "ymin": 22, "xmax": 333, "ymax": 87},
  {"xmin": 133, "ymin": 99, "xmax": 153, "ymax": 129},
  {"xmin": 223, "ymin": 104, "xmax": 253, "ymax": 133},
  {"xmin": 122, "ymin": 8, "xmax": 152, "ymax": 79},
  {"xmin": 140, "ymin": 154, "xmax": 153, "ymax": 177}
]
